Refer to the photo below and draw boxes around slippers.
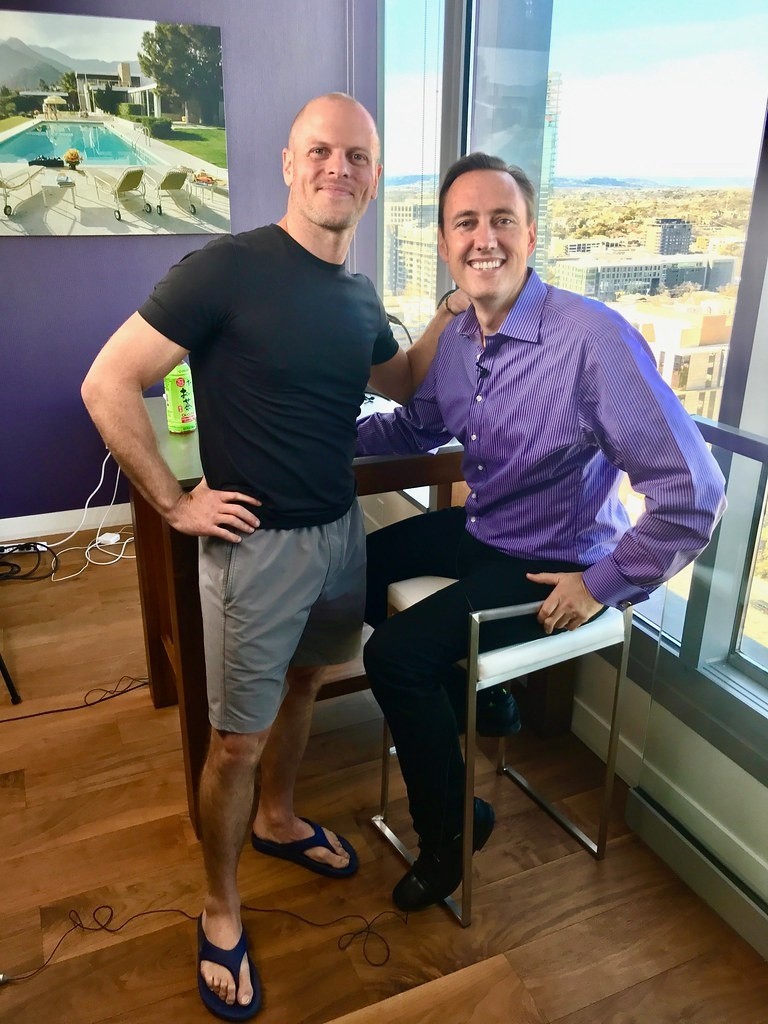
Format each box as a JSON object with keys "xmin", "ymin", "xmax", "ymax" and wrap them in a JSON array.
[
  {"xmin": 252, "ymin": 818, "xmax": 359, "ymax": 879},
  {"xmin": 197, "ymin": 912, "xmax": 261, "ymax": 1023}
]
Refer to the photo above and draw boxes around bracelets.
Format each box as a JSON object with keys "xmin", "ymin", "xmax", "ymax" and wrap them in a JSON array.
[{"xmin": 445, "ymin": 294, "xmax": 458, "ymax": 317}]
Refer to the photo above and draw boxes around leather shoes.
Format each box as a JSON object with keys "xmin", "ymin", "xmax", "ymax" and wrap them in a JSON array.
[
  {"xmin": 457, "ymin": 686, "xmax": 522, "ymax": 737},
  {"xmin": 393, "ymin": 796, "xmax": 495, "ymax": 911}
]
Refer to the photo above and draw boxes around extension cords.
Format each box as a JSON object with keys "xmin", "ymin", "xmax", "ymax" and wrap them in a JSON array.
[{"xmin": 0, "ymin": 542, "xmax": 49, "ymax": 551}]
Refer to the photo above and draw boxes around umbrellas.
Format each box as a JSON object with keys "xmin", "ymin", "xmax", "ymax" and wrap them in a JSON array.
[{"xmin": 44, "ymin": 95, "xmax": 67, "ymax": 105}]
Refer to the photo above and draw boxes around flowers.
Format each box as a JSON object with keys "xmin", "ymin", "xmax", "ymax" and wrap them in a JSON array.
[{"xmin": 63, "ymin": 147, "xmax": 83, "ymax": 166}]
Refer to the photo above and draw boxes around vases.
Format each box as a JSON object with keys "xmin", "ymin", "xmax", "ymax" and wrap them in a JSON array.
[{"xmin": 69, "ymin": 162, "xmax": 76, "ymax": 169}]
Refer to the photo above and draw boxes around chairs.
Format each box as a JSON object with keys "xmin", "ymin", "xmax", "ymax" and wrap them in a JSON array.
[
  {"xmin": 142, "ymin": 166, "xmax": 196, "ymax": 216},
  {"xmin": 94, "ymin": 165, "xmax": 151, "ymax": 221},
  {"xmin": 0, "ymin": 164, "xmax": 52, "ymax": 216}
]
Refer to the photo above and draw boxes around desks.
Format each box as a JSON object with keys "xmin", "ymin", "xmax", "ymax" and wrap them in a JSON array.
[
  {"xmin": 128, "ymin": 392, "xmax": 472, "ymax": 843},
  {"xmin": 41, "ymin": 174, "xmax": 78, "ymax": 207},
  {"xmin": 189, "ymin": 178, "xmax": 218, "ymax": 205}
]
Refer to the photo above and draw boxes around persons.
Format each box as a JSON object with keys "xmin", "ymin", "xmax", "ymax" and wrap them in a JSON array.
[
  {"xmin": 43, "ymin": 102, "xmax": 58, "ymax": 120},
  {"xmin": 81, "ymin": 92, "xmax": 470, "ymax": 1023},
  {"xmin": 355, "ymin": 153, "xmax": 729, "ymax": 911},
  {"xmin": 79, "ymin": 107, "xmax": 90, "ymax": 118}
]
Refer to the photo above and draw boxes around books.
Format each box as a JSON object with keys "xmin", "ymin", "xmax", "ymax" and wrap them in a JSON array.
[{"xmin": 56, "ymin": 175, "xmax": 76, "ymax": 188}]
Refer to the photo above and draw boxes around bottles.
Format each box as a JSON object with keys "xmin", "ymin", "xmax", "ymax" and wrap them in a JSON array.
[{"xmin": 164, "ymin": 359, "xmax": 196, "ymax": 435}]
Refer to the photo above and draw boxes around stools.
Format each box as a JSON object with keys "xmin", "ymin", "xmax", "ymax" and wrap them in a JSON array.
[{"xmin": 369, "ymin": 575, "xmax": 634, "ymax": 929}]
